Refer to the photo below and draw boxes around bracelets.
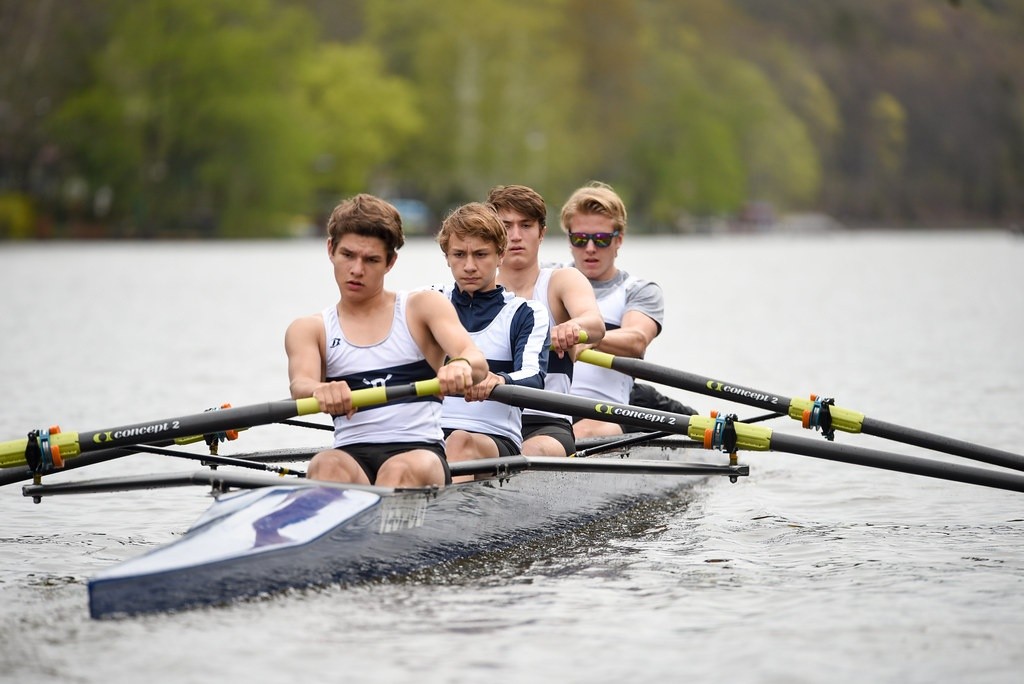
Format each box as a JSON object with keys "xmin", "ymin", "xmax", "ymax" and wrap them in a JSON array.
[
  {"xmin": 497, "ymin": 372, "xmax": 512, "ymax": 384},
  {"xmin": 445, "ymin": 358, "xmax": 470, "ymax": 366}
]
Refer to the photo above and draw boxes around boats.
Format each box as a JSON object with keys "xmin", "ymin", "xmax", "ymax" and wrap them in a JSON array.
[{"xmin": 87, "ymin": 426, "xmax": 724, "ymax": 619}]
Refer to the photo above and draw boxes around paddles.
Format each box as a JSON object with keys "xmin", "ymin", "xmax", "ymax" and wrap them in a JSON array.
[
  {"xmin": 450, "ymin": 383, "xmax": 1024, "ymax": 495},
  {"xmin": 574, "ymin": 349, "xmax": 1024, "ymax": 475},
  {"xmin": 24, "ymin": 328, "xmax": 589, "ymax": 478}
]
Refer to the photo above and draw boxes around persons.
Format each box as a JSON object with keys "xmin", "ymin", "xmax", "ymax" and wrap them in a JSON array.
[
  {"xmin": 541, "ymin": 181, "xmax": 664, "ymax": 440},
  {"xmin": 285, "ymin": 193, "xmax": 488, "ymax": 487},
  {"xmin": 487, "ymin": 184, "xmax": 606, "ymax": 457},
  {"xmin": 414, "ymin": 202, "xmax": 551, "ymax": 483}
]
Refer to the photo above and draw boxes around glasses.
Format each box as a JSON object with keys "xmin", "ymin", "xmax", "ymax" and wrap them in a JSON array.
[{"xmin": 568, "ymin": 227, "xmax": 618, "ymax": 248}]
